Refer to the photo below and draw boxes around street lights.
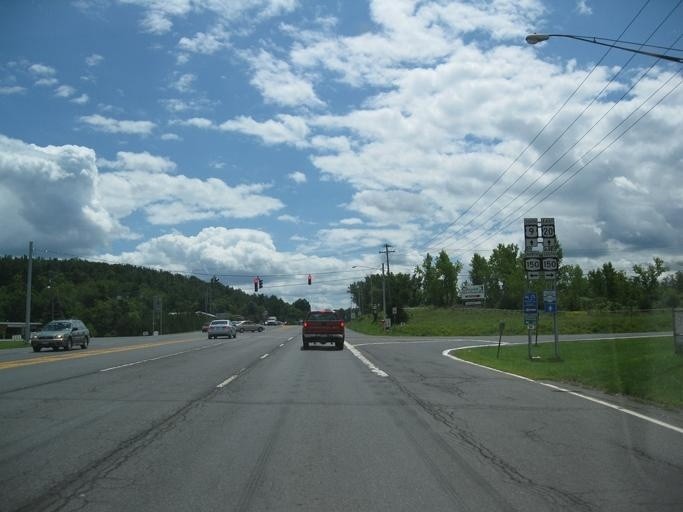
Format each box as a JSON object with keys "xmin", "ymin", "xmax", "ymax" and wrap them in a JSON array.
[
  {"xmin": 524, "ymin": 33, "xmax": 682, "ymax": 65},
  {"xmin": 351, "ymin": 262, "xmax": 388, "ymax": 326}
]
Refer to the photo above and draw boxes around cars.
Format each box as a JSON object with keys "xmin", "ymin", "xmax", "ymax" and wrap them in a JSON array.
[
  {"xmin": 201, "ymin": 318, "xmax": 265, "ymax": 339},
  {"xmin": 28, "ymin": 318, "xmax": 91, "ymax": 354}
]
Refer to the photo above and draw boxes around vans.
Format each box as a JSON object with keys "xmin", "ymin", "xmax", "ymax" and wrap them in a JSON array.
[{"xmin": 267, "ymin": 315, "xmax": 277, "ymax": 326}]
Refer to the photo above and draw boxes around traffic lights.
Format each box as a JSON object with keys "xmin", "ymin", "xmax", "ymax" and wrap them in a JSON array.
[
  {"xmin": 258, "ymin": 278, "xmax": 263, "ymax": 288},
  {"xmin": 254, "ymin": 279, "xmax": 258, "ymax": 292},
  {"xmin": 307, "ymin": 276, "xmax": 311, "ymax": 285},
  {"xmin": 214, "ymin": 278, "xmax": 219, "ymax": 288}
]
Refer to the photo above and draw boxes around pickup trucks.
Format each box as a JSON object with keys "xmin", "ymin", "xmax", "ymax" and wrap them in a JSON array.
[{"xmin": 301, "ymin": 311, "xmax": 345, "ymax": 351}]
